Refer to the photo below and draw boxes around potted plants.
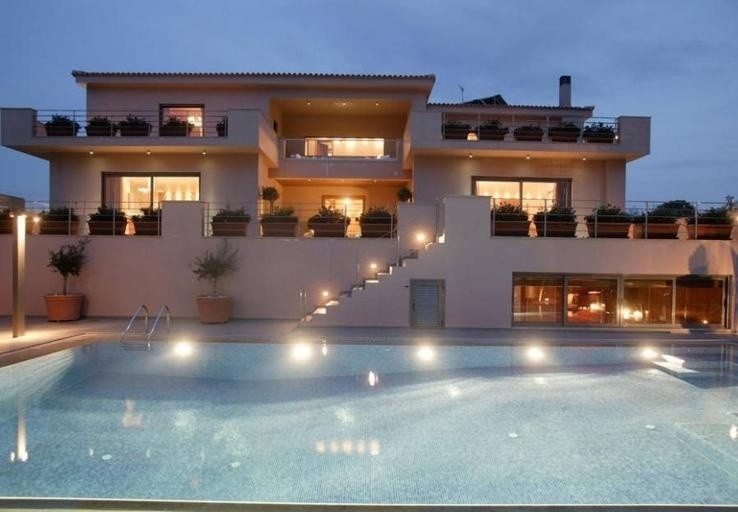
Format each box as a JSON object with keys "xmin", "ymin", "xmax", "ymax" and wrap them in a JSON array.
[
  {"xmin": 38, "ymin": 205, "xmax": 81, "ymax": 238},
  {"xmin": 215, "ymin": 116, "xmax": 227, "ymax": 137},
  {"xmin": 307, "ymin": 205, "xmax": 352, "ymax": 237},
  {"xmin": 42, "ymin": 112, "xmax": 195, "ymax": 137},
  {"xmin": 441, "ymin": 117, "xmax": 615, "ymax": 144},
  {"xmin": 86, "ymin": 205, "xmax": 128, "ymax": 236},
  {"xmin": 2, "ymin": 205, "xmax": 35, "ymax": 235},
  {"xmin": 210, "ymin": 206, "xmax": 250, "ymax": 237},
  {"xmin": 258, "ymin": 205, "xmax": 305, "ymax": 236},
  {"xmin": 491, "ymin": 199, "xmax": 734, "ymax": 238},
  {"xmin": 43, "ymin": 234, "xmax": 92, "ymax": 322},
  {"xmin": 130, "ymin": 205, "xmax": 162, "ymax": 235},
  {"xmin": 189, "ymin": 240, "xmax": 241, "ymax": 324},
  {"xmin": 359, "ymin": 205, "xmax": 398, "ymax": 237}
]
